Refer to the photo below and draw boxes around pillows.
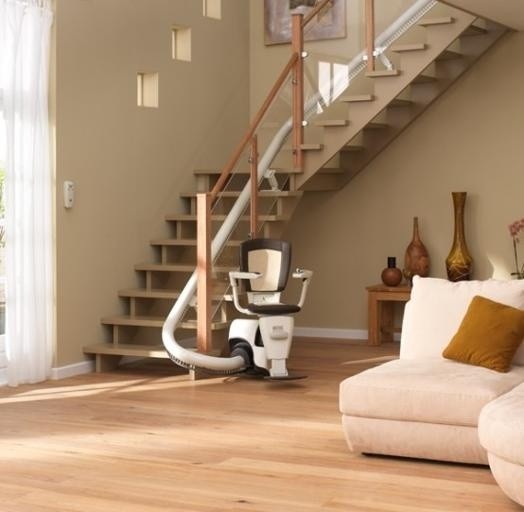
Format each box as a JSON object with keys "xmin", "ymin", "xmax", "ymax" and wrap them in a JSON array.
[{"xmin": 443, "ymin": 294, "xmax": 523, "ymax": 374}]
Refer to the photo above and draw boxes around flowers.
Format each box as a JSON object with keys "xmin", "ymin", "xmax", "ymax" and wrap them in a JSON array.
[{"xmin": 507, "ymin": 216, "xmax": 524, "ymax": 279}]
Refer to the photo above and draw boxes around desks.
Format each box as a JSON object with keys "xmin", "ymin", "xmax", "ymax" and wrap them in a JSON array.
[{"xmin": 364, "ymin": 286, "xmax": 411, "ymax": 346}]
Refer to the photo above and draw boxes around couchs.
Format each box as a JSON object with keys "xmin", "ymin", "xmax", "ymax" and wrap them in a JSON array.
[{"xmin": 339, "ymin": 275, "xmax": 524, "ymax": 506}]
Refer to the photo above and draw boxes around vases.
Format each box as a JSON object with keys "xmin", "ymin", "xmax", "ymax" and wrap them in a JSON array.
[
  {"xmin": 380, "ymin": 256, "xmax": 402, "ymax": 287},
  {"xmin": 445, "ymin": 191, "xmax": 473, "ymax": 282},
  {"xmin": 403, "ymin": 216, "xmax": 431, "ymax": 284}
]
